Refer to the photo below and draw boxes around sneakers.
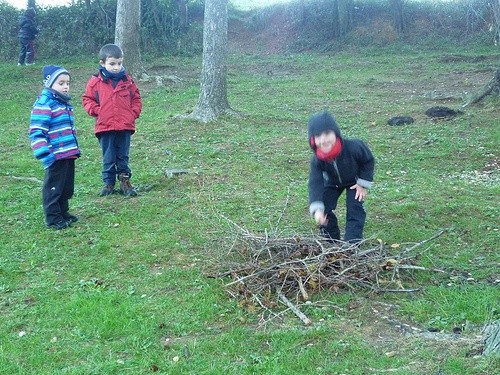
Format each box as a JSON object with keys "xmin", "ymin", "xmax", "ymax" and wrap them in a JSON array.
[
  {"xmin": 46, "ymin": 215, "xmax": 78, "ymax": 230},
  {"xmin": 101, "ymin": 184, "xmax": 115, "ymax": 195},
  {"xmin": 120, "ymin": 179, "xmax": 137, "ymax": 197}
]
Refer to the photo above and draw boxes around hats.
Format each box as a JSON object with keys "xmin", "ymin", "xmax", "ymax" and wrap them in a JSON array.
[{"xmin": 43, "ymin": 64, "xmax": 69, "ymax": 88}]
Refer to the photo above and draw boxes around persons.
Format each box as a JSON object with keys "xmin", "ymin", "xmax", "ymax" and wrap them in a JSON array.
[
  {"xmin": 28, "ymin": 64, "xmax": 82, "ymax": 230},
  {"xmin": 307, "ymin": 110, "xmax": 376, "ymax": 248},
  {"xmin": 82, "ymin": 44, "xmax": 143, "ymax": 197},
  {"xmin": 17, "ymin": 7, "xmax": 40, "ymax": 66}
]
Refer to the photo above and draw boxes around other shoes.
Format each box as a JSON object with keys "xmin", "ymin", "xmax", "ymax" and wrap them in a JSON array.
[
  {"xmin": 26, "ymin": 63, "xmax": 35, "ymax": 65},
  {"xmin": 18, "ymin": 63, "xmax": 21, "ymax": 65}
]
